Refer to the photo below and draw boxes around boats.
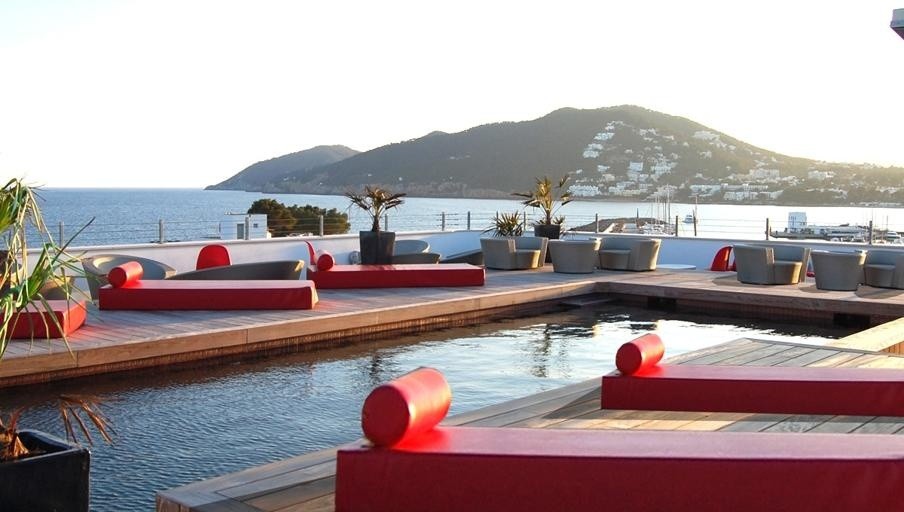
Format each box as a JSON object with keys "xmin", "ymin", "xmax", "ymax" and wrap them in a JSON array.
[
  {"xmin": 764, "ymin": 212, "xmax": 903, "ymax": 241},
  {"xmin": 684, "ymin": 213, "xmax": 693, "ymax": 223}
]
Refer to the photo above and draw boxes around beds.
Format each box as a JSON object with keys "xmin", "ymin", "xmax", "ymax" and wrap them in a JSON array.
[
  {"xmin": 338, "ymin": 367, "xmax": 903, "ymax": 511},
  {"xmin": 601, "ymin": 334, "xmax": 904, "ymax": 412},
  {"xmin": 316, "ymin": 252, "xmax": 487, "ymax": 287},
  {"xmin": 0, "ymin": 294, "xmax": 88, "ymax": 339},
  {"xmin": 98, "ymin": 260, "xmax": 317, "ymax": 311}
]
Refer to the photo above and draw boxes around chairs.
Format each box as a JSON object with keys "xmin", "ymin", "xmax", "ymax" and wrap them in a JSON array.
[
  {"xmin": 304, "ymin": 238, "xmax": 319, "ymax": 265},
  {"xmin": 705, "ymin": 245, "xmax": 737, "ymax": 271},
  {"xmin": 597, "ymin": 235, "xmax": 662, "ymax": 272},
  {"xmin": 730, "ymin": 238, "xmax": 810, "ymax": 284},
  {"xmin": 392, "ymin": 236, "xmax": 549, "ymax": 270},
  {"xmin": 10, "ymin": 255, "xmax": 177, "ymax": 300},
  {"xmin": 195, "ymin": 244, "xmax": 231, "ymax": 269},
  {"xmin": 862, "ymin": 249, "xmax": 904, "ymax": 290}
]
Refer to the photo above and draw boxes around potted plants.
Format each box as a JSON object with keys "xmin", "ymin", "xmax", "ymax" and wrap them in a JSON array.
[
  {"xmin": 0, "ymin": 177, "xmax": 116, "ymax": 511},
  {"xmin": 511, "ymin": 172, "xmax": 573, "ymax": 234}
]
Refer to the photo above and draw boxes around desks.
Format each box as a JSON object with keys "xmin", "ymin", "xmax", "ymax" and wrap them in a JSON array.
[
  {"xmin": 812, "ymin": 249, "xmax": 866, "ymax": 292},
  {"xmin": 548, "ymin": 240, "xmax": 596, "ymax": 274},
  {"xmin": 656, "ymin": 263, "xmax": 696, "ymax": 271}
]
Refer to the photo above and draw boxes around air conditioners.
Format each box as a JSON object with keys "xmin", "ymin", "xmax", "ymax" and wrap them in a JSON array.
[{"xmin": 342, "ymin": 182, "xmax": 405, "ymax": 264}]
